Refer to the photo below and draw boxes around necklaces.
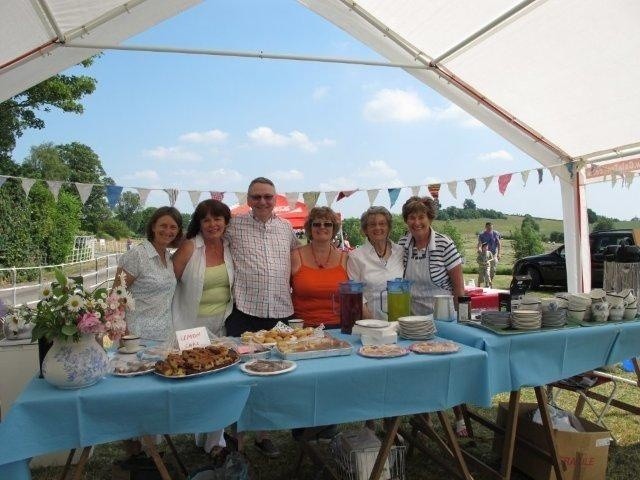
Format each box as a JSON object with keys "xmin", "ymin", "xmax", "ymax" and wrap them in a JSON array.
[
  {"xmin": 310, "ymin": 243, "xmax": 331, "ymax": 269},
  {"xmin": 372, "ymin": 241, "xmax": 387, "ymax": 265},
  {"xmin": 412, "ymin": 238, "xmax": 429, "ymax": 259}
]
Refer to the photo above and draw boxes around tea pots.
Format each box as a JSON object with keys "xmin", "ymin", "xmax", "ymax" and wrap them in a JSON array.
[
  {"xmin": 433, "ymin": 294, "xmax": 455, "ymax": 323},
  {"xmin": 379, "ymin": 277, "xmax": 416, "ymax": 324},
  {"xmin": 331, "ymin": 278, "xmax": 368, "ymax": 334}
]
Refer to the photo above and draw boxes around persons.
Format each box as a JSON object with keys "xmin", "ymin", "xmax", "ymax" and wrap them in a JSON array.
[
  {"xmin": 171, "ymin": 199, "xmax": 235, "ymax": 457},
  {"xmin": 338, "ymin": 232, "xmax": 353, "ymax": 252},
  {"xmin": 476, "ymin": 222, "xmax": 501, "ymax": 288},
  {"xmin": 347, "ymin": 206, "xmax": 404, "ymax": 445},
  {"xmin": 225, "ymin": 178, "xmax": 303, "ymax": 456},
  {"xmin": 108, "ymin": 205, "xmax": 183, "ymax": 446},
  {"xmin": 126, "ymin": 236, "xmax": 132, "ymax": 251},
  {"xmin": 398, "ymin": 197, "xmax": 470, "ymax": 436},
  {"xmin": 289, "ymin": 206, "xmax": 347, "ymax": 447},
  {"xmin": 295, "ymin": 231, "xmax": 304, "ymax": 238}
]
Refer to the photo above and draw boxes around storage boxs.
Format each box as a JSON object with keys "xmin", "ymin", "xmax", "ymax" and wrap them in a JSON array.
[{"xmin": 492, "ymin": 403, "xmax": 612, "ymax": 480}]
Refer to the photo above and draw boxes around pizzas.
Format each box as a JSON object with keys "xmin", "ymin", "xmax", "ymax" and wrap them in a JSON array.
[
  {"xmin": 413, "ymin": 341, "xmax": 457, "ymax": 352},
  {"xmin": 246, "ymin": 359, "xmax": 291, "ymax": 372},
  {"xmin": 358, "ymin": 344, "xmax": 408, "ymax": 357}
]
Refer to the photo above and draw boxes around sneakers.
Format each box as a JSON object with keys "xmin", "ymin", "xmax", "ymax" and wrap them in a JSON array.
[{"xmin": 254, "ymin": 436, "xmax": 280, "ymax": 458}]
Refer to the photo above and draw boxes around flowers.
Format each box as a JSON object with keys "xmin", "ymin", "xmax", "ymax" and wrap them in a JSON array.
[{"xmin": 6, "ymin": 269, "xmax": 136, "ymax": 344}]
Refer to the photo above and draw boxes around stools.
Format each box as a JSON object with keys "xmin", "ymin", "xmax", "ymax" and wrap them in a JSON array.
[{"xmin": 548, "ymin": 370, "xmax": 617, "ymax": 441}]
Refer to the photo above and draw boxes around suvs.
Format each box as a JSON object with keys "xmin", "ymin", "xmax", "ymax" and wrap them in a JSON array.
[{"xmin": 511, "ymin": 229, "xmax": 638, "ymax": 289}]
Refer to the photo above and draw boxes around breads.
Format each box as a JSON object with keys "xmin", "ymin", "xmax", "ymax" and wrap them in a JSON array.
[
  {"xmin": 115, "ymin": 360, "xmax": 148, "ymax": 372},
  {"xmin": 241, "ymin": 327, "xmax": 351, "ymax": 353},
  {"xmin": 155, "ymin": 345, "xmax": 238, "ymax": 376}
]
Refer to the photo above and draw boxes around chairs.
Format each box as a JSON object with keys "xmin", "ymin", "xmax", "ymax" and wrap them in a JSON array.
[{"xmin": 499, "ymin": 274, "xmax": 532, "ymax": 312}]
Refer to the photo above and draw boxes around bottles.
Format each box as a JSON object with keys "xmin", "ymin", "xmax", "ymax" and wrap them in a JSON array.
[
  {"xmin": 467, "ymin": 279, "xmax": 475, "ymax": 291},
  {"xmin": 223, "ymin": 451, "xmax": 249, "ymax": 480}
]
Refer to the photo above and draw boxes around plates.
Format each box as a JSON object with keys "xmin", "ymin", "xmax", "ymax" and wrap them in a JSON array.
[
  {"xmin": 480, "ymin": 308, "xmax": 542, "ymax": 330},
  {"xmin": 117, "ymin": 344, "xmax": 148, "ymax": 354},
  {"xmin": 359, "ymin": 344, "xmax": 411, "ymax": 358},
  {"xmin": 235, "ymin": 347, "xmax": 273, "ymax": 363},
  {"xmin": 396, "ymin": 314, "xmax": 436, "ymax": 341},
  {"xmin": 354, "ymin": 319, "xmax": 390, "ymax": 329},
  {"xmin": 237, "ymin": 359, "xmax": 297, "ymax": 376},
  {"xmin": 409, "ymin": 340, "xmax": 461, "ymax": 355},
  {"xmin": 105, "ymin": 361, "xmax": 156, "ymax": 376},
  {"xmin": 152, "ymin": 353, "xmax": 242, "ymax": 379}
]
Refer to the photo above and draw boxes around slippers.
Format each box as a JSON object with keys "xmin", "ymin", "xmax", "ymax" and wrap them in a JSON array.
[{"xmin": 456, "ymin": 419, "xmax": 468, "ymax": 437}]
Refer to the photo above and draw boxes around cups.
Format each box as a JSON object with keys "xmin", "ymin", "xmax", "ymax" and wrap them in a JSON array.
[
  {"xmin": 520, "ymin": 287, "xmax": 639, "ymax": 327},
  {"xmin": 287, "ymin": 319, "xmax": 304, "ymax": 329},
  {"xmin": 121, "ymin": 334, "xmax": 142, "ymax": 351}
]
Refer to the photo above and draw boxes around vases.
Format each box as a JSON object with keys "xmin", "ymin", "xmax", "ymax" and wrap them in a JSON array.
[{"xmin": 42, "ymin": 333, "xmax": 110, "ymax": 390}]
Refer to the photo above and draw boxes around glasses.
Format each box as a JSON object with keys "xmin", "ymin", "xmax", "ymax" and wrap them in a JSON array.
[
  {"xmin": 313, "ymin": 223, "xmax": 334, "ymax": 227},
  {"xmin": 248, "ymin": 194, "xmax": 275, "ymax": 200}
]
